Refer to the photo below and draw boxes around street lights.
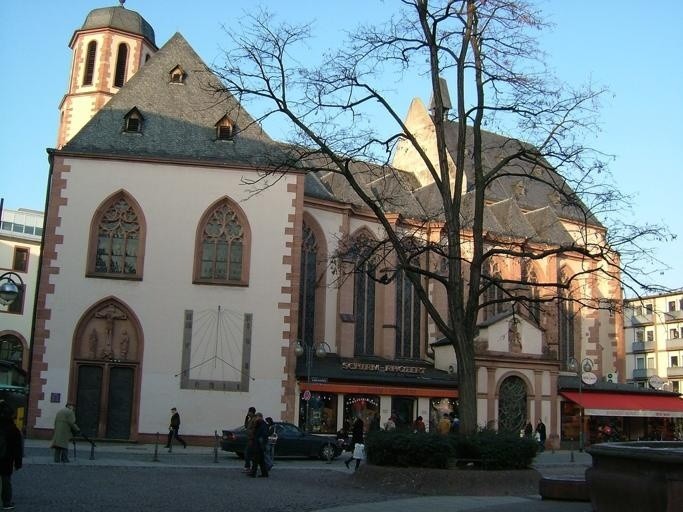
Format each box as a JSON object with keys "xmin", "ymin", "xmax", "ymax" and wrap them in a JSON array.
[
  {"xmin": 0, "ymin": 271, "xmax": 24, "ymax": 307},
  {"xmin": 289, "ymin": 338, "xmax": 329, "ymax": 434},
  {"xmin": 568, "ymin": 355, "xmax": 593, "ymax": 453}
]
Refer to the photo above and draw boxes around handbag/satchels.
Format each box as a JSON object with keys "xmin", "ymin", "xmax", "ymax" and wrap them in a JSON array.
[{"xmin": 353, "ymin": 442, "xmax": 365, "ymax": 460}]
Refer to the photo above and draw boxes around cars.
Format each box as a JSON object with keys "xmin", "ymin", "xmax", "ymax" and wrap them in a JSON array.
[{"xmin": 221, "ymin": 421, "xmax": 342, "ymax": 459}]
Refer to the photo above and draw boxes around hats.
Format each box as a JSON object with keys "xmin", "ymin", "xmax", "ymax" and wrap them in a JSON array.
[{"xmin": 253, "ymin": 412, "xmax": 262, "ymax": 417}]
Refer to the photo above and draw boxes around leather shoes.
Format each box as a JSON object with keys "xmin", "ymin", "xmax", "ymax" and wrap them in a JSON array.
[{"xmin": 344, "ymin": 460, "xmax": 350, "ymax": 469}]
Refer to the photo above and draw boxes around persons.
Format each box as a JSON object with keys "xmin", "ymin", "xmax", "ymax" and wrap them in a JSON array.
[
  {"xmin": 240, "ymin": 407, "xmax": 276, "ymax": 478},
  {"xmin": 164, "ymin": 407, "xmax": 187, "ymax": 449},
  {"xmin": 336, "ymin": 413, "xmax": 547, "ymax": 478},
  {"xmin": 637, "ymin": 421, "xmax": 663, "ymax": 441},
  {"xmin": 49, "ymin": 401, "xmax": 81, "ymax": 463},
  {"xmin": 0, "ymin": 399, "xmax": 24, "ymax": 510}
]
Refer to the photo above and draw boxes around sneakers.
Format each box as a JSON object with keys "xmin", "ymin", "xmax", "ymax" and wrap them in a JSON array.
[
  {"xmin": 247, "ymin": 473, "xmax": 257, "ymax": 478},
  {"xmin": 258, "ymin": 475, "xmax": 268, "ymax": 479}
]
